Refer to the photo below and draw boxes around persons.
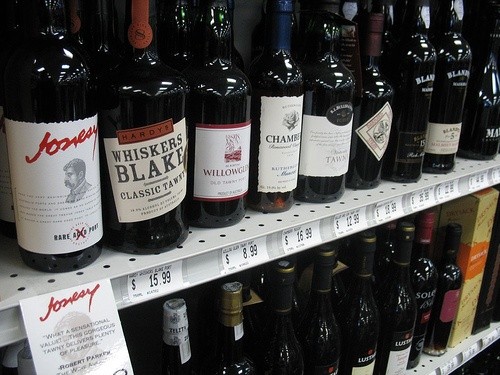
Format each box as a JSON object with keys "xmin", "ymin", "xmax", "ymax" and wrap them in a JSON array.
[{"xmin": 63, "ymin": 159, "xmax": 92, "ymax": 203}]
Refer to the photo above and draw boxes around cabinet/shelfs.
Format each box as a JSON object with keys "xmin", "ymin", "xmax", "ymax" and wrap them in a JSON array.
[{"xmin": 0, "ymin": 152, "xmax": 500, "ymax": 375}]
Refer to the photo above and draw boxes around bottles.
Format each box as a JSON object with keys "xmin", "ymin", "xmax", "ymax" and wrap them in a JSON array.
[
  {"xmin": 304, "ymin": 229, "xmax": 378, "ymax": 375},
  {"xmin": 158, "ymin": 299, "xmax": 202, "ymax": 375},
  {"xmin": 0, "ymin": 0, "xmax": 103, "ymax": 273},
  {"xmin": 247, "ymin": 0, "xmax": 304, "ymax": 213},
  {"xmin": 3, "ymin": 339, "xmax": 36, "ymax": 375},
  {"xmin": 94, "ymin": 0, "xmax": 189, "ymax": 255},
  {"xmin": 423, "ymin": 0, "xmax": 500, "ymax": 174},
  {"xmin": 374, "ymin": 210, "xmax": 462, "ymax": 375},
  {"xmin": 296, "ymin": 0, "xmax": 355, "ymax": 203},
  {"xmin": 183, "ymin": 0, "xmax": 251, "ymax": 228},
  {"xmin": 346, "ymin": 0, "xmax": 437, "ymax": 190},
  {"xmin": 206, "ymin": 257, "xmax": 304, "ymax": 375},
  {"xmin": 458, "ymin": 338, "xmax": 500, "ymax": 375}
]
